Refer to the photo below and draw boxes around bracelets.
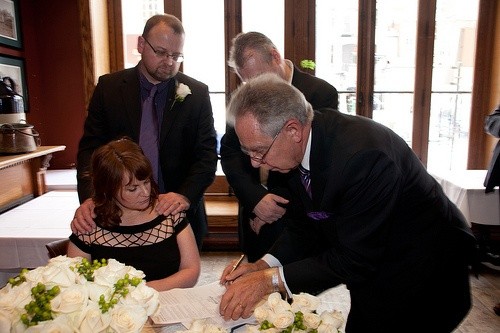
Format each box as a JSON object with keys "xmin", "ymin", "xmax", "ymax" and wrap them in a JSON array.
[{"xmin": 271, "ymin": 267, "xmax": 280, "ymax": 292}]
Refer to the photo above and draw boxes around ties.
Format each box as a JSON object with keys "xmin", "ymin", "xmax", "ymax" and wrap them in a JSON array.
[
  {"xmin": 298, "ymin": 166, "xmax": 312, "ymax": 199},
  {"xmin": 138, "ymin": 83, "xmax": 158, "ymax": 188}
]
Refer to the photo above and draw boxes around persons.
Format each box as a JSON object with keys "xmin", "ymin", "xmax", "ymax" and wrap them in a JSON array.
[
  {"xmin": 67, "ymin": 140, "xmax": 201, "ymax": 292},
  {"xmin": 69, "ymin": 15, "xmax": 219, "ymax": 246},
  {"xmin": 482, "ymin": 104, "xmax": 500, "ymax": 318},
  {"xmin": 220, "ymin": 32, "xmax": 337, "ymax": 266},
  {"xmin": 219, "ymin": 71, "xmax": 480, "ymax": 333}
]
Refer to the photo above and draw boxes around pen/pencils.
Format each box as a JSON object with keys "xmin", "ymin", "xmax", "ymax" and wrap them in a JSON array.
[{"xmin": 225, "ymin": 254, "xmax": 244, "ymax": 283}]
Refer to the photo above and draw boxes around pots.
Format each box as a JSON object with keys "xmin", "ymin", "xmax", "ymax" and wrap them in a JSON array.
[{"xmin": 0, "ymin": 119, "xmax": 39, "ymax": 154}]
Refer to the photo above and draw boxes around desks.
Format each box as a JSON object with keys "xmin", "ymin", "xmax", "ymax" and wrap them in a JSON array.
[
  {"xmin": 428, "ymin": 169, "xmax": 500, "ymax": 279},
  {"xmin": 0, "ymin": 190, "xmax": 80, "ymax": 288},
  {"xmin": 44, "ymin": 169, "xmax": 77, "ymax": 191},
  {"xmin": 0, "ymin": 145, "xmax": 66, "ymax": 214}
]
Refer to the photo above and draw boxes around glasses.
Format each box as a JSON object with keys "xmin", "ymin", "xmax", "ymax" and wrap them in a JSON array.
[
  {"xmin": 144, "ymin": 38, "xmax": 185, "ymax": 64},
  {"xmin": 242, "ymin": 119, "xmax": 290, "ymax": 165}
]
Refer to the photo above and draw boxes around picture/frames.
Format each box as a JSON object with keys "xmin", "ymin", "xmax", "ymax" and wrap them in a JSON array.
[
  {"xmin": 0, "ymin": 0, "xmax": 24, "ymax": 52},
  {"xmin": 0, "ymin": 53, "xmax": 30, "ymax": 113}
]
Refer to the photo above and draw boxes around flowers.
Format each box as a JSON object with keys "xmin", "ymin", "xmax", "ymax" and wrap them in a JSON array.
[
  {"xmin": 0, "ymin": 254, "xmax": 161, "ymax": 333},
  {"xmin": 170, "ymin": 82, "xmax": 192, "ymax": 111},
  {"xmin": 246, "ymin": 291, "xmax": 344, "ymax": 333},
  {"xmin": 175, "ymin": 318, "xmax": 228, "ymax": 333}
]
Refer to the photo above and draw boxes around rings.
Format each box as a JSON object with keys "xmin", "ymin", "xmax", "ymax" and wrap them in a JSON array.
[
  {"xmin": 74, "ymin": 216, "xmax": 77, "ymax": 219},
  {"xmin": 238, "ymin": 303, "xmax": 245, "ymax": 308}
]
